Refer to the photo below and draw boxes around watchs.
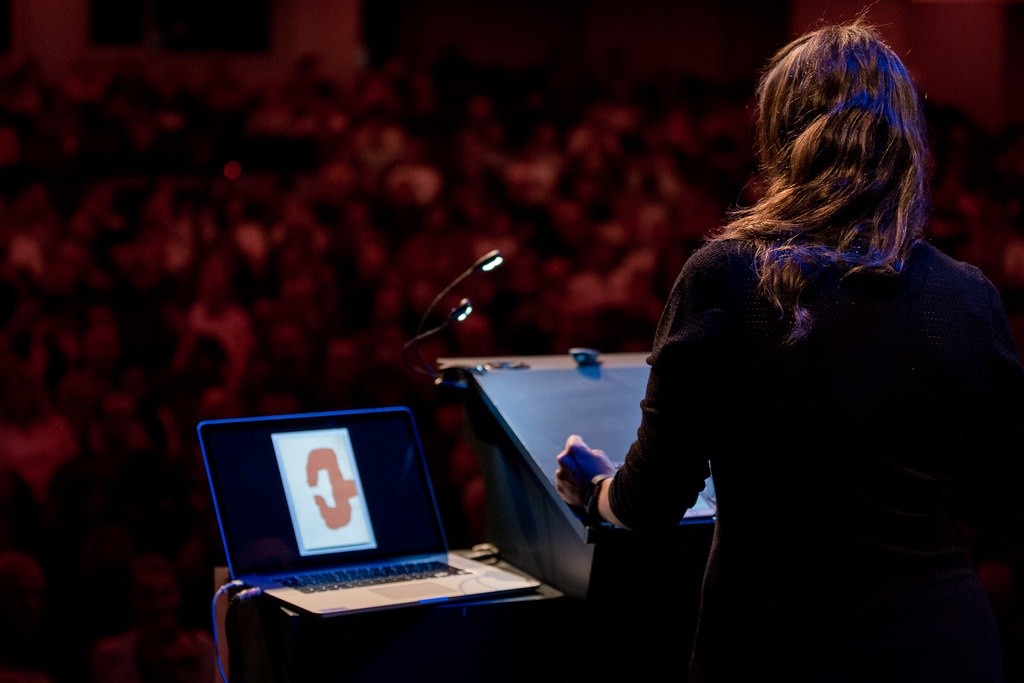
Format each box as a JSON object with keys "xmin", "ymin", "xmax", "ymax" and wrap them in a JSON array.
[{"xmin": 583, "ymin": 474, "xmax": 611, "ymax": 521}]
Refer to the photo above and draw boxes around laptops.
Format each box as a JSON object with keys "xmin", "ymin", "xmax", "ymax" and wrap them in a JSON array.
[{"xmin": 197, "ymin": 406, "xmax": 540, "ymax": 619}]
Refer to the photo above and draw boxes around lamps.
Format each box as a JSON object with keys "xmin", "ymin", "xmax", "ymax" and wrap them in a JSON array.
[{"xmin": 400, "ymin": 247, "xmax": 506, "ymax": 389}]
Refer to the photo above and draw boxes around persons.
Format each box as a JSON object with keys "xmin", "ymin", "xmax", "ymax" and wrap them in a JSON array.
[
  {"xmin": 557, "ymin": 12, "xmax": 1024, "ymax": 683},
  {"xmin": 0, "ymin": 45, "xmax": 761, "ymax": 683},
  {"xmin": 923, "ymin": 98, "xmax": 1024, "ymax": 362}
]
